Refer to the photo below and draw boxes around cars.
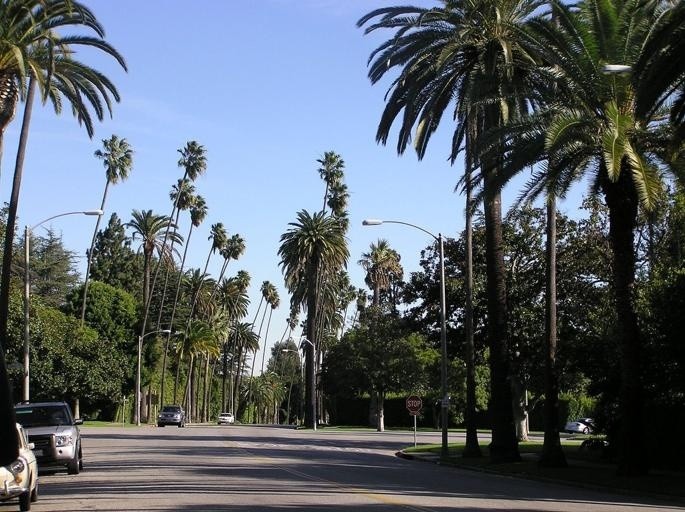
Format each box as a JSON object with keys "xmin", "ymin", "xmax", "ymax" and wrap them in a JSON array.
[
  {"xmin": 0, "ymin": 423, "xmax": 38, "ymax": 512},
  {"xmin": 564, "ymin": 418, "xmax": 596, "ymax": 435},
  {"xmin": 218, "ymin": 413, "xmax": 234, "ymax": 425}
]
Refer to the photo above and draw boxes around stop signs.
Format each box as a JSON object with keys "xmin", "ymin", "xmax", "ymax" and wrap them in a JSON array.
[{"xmin": 406, "ymin": 395, "xmax": 423, "ymax": 414}]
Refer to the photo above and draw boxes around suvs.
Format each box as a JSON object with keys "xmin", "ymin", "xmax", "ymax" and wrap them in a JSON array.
[
  {"xmin": 158, "ymin": 405, "xmax": 186, "ymax": 427},
  {"xmin": 14, "ymin": 400, "xmax": 83, "ymax": 474}
]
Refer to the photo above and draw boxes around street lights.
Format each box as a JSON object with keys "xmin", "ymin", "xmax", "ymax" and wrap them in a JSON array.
[
  {"xmin": 363, "ymin": 219, "xmax": 448, "ymax": 461},
  {"xmin": 282, "ymin": 336, "xmax": 317, "ymax": 431},
  {"xmin": 136, "ymin": 330, "xmax": 172, "ymax": 427},
  {"xmin": 23, "ymin": 208, "xmax": 105, "ymax": 404}
]
